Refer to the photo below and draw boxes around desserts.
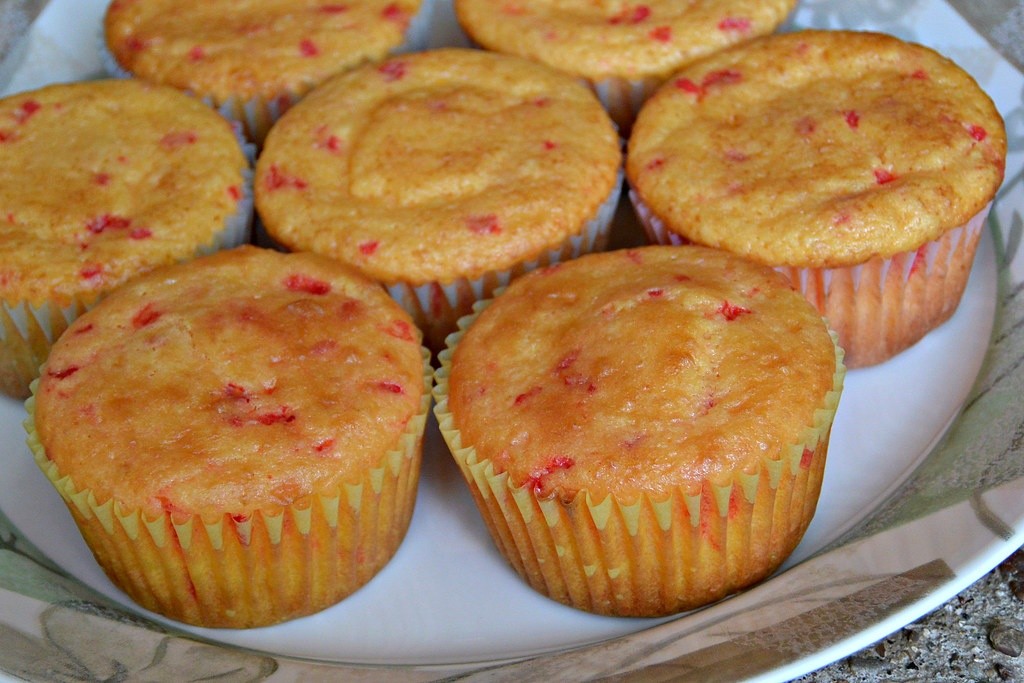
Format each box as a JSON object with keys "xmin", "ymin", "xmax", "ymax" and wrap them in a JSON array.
[{"xmin": 0, "ymin": 0, "xmax": 1008, "ymax": 626}]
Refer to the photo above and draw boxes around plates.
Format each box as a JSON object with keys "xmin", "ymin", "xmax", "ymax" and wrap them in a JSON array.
[{"xmin": 0, "ymin": 0, "xmax": 1024, "ymax": 683}]
